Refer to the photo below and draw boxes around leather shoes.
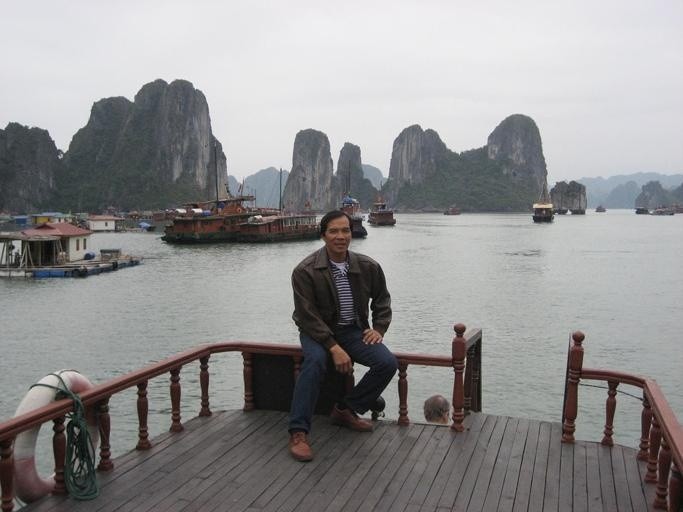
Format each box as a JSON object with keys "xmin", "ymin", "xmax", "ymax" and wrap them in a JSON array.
[
  {"xmin": 330, "ymin": 403, "xmax": 375, "ymax": 432},
  {"xmin": 290, "ymin": 432, "xmax": 314, "ymax": 461}
]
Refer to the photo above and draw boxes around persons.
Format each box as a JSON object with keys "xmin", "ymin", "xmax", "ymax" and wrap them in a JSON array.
[
  {"xmin": 425, "ymin": 393, "xmax": 451, "ymax": 425},
  {"xmin": 287, "ymin": 211, "xmax": 399, "ymax": 462}
]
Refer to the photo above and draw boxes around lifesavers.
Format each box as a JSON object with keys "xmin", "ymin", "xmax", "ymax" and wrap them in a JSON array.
[{"xmin": 11, "ymin": 370, "xmax": 98, "ymax": 504}]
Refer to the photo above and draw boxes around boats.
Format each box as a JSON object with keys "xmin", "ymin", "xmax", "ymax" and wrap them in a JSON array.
[
  {"xmin": 529, "ymin": 201, "xmax": 585, "ymax": 225},
  {"xmin": 157, "ymin": 156, "xmax": 368, "ymax": 246},
  {"xmin": 593, "ymin": 205, "xmax": 607, "ymax": 212},
  {"xmin": 631, "ymin": 202, "xmax": 682, "ymax": 216},
  {"xmin": 366, "ymin": 194, "xmax": 397, "ymax": 227},
  {"xmin": 442, "ymin": 205, "xmax": 461, "ymax": 216}
]
